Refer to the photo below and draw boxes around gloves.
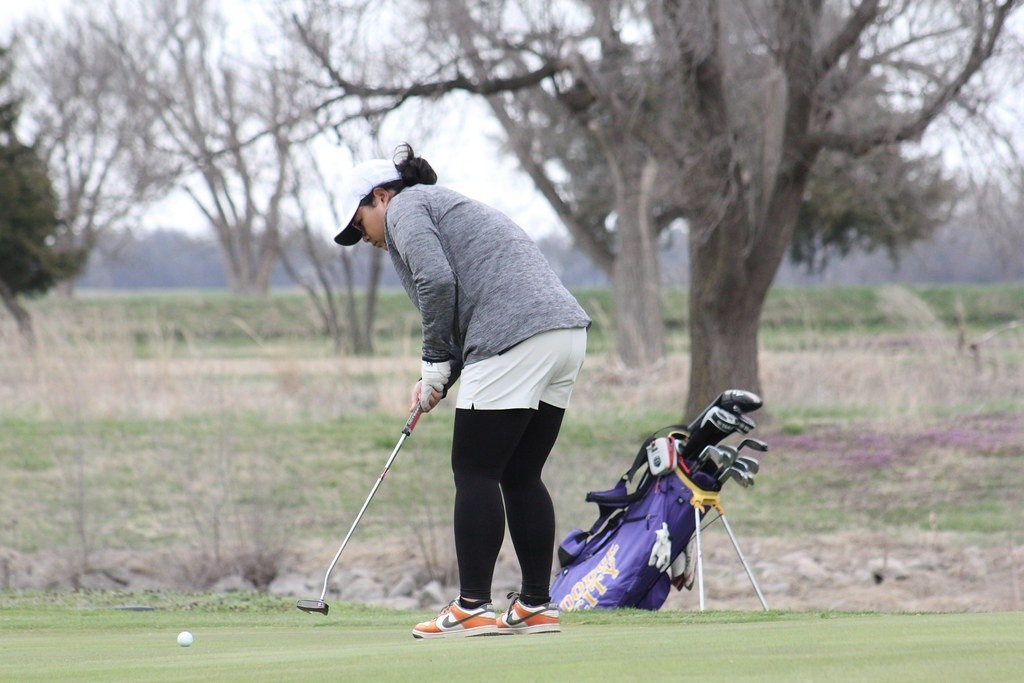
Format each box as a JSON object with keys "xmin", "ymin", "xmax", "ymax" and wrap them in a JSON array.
[{"xmin": 420, "ymin": 357, "xmax": 451, "ymax": 412}]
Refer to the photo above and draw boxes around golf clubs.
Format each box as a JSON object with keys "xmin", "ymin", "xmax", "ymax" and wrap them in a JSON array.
[
  {"xmin": 686, "ymin": 390, "xmax": 769, "ymax": 487},
  {"xmin": 297, "ymin": 403, "xmax": 423, "ymax": 615}
]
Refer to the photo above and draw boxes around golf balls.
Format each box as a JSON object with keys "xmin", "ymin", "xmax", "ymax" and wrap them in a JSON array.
[{"xmin": 177, "ymin": 630, "xmax": 193, "ymax": 646}]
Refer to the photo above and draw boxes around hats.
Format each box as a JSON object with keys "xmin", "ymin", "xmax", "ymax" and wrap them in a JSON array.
[{"xmin": 333, "ymin": 158, "xmax": 405, "ymax": 245}]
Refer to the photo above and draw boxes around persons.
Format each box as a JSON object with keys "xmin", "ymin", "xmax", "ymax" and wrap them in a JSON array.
[{"xmin": 332, "ymin": 141, "xmax": 591, "ymax": 640}]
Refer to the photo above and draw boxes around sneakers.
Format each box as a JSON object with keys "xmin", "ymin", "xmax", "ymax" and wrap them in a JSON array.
[
  {"xmin": 497, "ymin": 597, "xmax": 561, "ymax": 634},
  {"xmin": 413, "ymin": 596, "xmax": 499, "ymax": 638}
]
